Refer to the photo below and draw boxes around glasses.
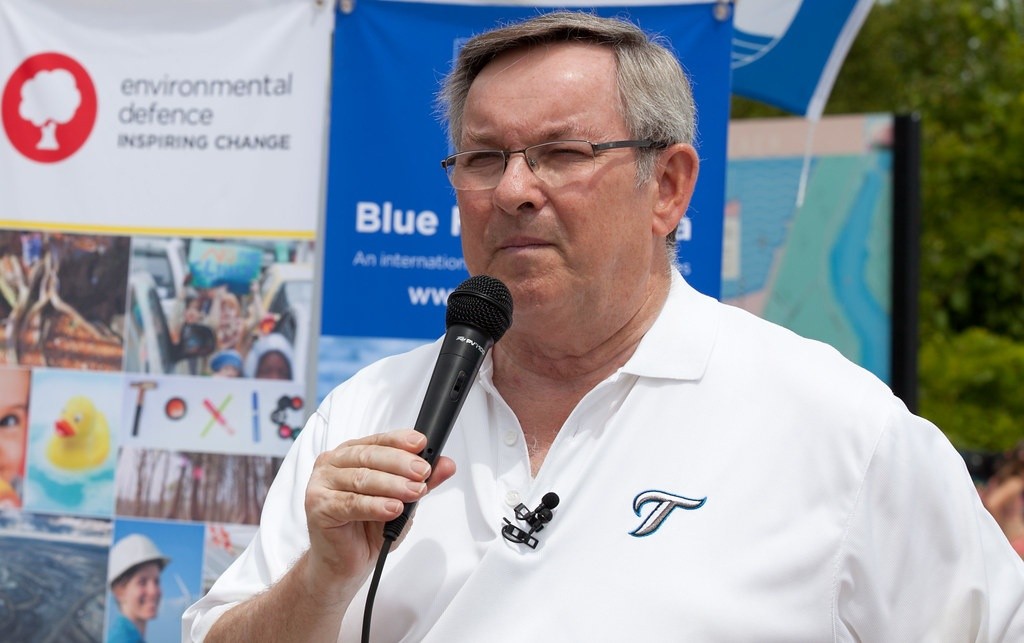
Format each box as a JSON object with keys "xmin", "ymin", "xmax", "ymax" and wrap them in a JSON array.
[{"xmin": 441, "ymin": 136, "xmax": 668, "ymax": 191}]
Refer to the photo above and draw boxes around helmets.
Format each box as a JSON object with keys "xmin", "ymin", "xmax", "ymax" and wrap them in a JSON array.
[{"xmin": 107, "ymin": 534, "xmax": 166, "ymax": 587}]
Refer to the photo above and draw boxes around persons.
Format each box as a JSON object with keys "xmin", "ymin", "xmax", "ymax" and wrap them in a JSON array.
[
  {"xmin": 0, "ymin": 367, "xmax": 31, "ymax": 500},
  {"xmin": 105, "ymin": 534, "xmax": 172, "ymax": 643},
  {"xmin": 179, "ymin": 9, "xmax": 1024, "ymax": 643},
  {"xmin": 186, "ymin": 292, "xmax": 295, "ymax": 379},
  {"xmin": 981, "ymin": 448, "xmax": 1024, "ymax": 560}
]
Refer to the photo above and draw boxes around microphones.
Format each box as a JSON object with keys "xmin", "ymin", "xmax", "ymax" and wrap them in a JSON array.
[
  {"xmin": 527, "ymin": 493, "xmax": 560, "ymax": 537},
  {"xmin": 384, "ymin": 275, "xmax": 515, "ymax": 539}
]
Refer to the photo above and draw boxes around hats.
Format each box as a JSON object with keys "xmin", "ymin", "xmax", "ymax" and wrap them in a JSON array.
[{"xmin": 243, "ymin": 331, "xmax": 295, "ymax": 379}]
